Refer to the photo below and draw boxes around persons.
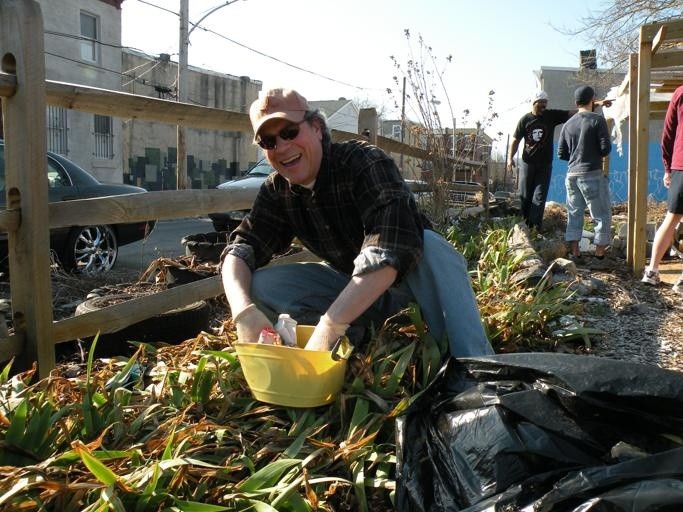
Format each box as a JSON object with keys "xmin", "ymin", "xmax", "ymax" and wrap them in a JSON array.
[
  {"xmin": 556, "ymin": 83, "xmax": 611, "ymax": 263},
  {"xmin": 505, "ymin": 89, "xmax": 616, "ymax": 237},
  {"xmin": 217, "ymin": 88, "xmax": 495, "ymax": 396},
  {"xmin": 638, "ymin": 86, "xmax": 682, "ymax": 296}
]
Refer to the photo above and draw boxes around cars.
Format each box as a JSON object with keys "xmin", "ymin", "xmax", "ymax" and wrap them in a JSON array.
[
  {"xmin": 1, "ymin": 138, "xmax": 161, "ymax": 283},
  {"xmin": 402, "ymin": 178, "xmax": 514, "ymax": 210},
  {"xmin": 205, "ymin": 153, "xmax": 279, "ymax": 231}
]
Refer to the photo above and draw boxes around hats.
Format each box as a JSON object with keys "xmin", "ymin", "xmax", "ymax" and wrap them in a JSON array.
[
  {"xmin": 531, "ymin": 89, "xmax": 548, "ymax": 104},
  {"xmin": 249, "ymin": 87, "xmax": 309, "ymax": 144},
  {"xmin": 574, "ymin": 86, "xmax": 594, "ymax": 103}
]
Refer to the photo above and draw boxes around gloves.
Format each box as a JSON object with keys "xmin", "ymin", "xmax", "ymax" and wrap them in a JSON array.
[
  {"xmin": 234, "ymin": 302, "xmax": 274, "ymax": 344},
  {"xmin": 305, "ymin": 312, "xmax": 351, "ymax": 351}
]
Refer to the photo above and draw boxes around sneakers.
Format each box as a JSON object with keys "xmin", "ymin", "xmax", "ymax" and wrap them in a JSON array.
[
  {"xmin": 537, "ymin": 229, "xmax": 557, "ymax": 241},
  {"xmin": 569, "ymin": 253, "xmax": 591, "ymax": 265},
  {"xmin": 590, "ymin": 255, "xmax": 617, "ymax": 270},
  {"xmin": 640, "ymin": 268, "xmax": 659, "ymax": 286},
  {"xmin": 671, "ymin": 276, "xmax": 683, "ymax": 295}
]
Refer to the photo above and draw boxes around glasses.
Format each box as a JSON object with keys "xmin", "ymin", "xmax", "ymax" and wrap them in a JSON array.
[{"xmin": 257, "ymin": 118, "xmax": 308, "ymax": 149}]
